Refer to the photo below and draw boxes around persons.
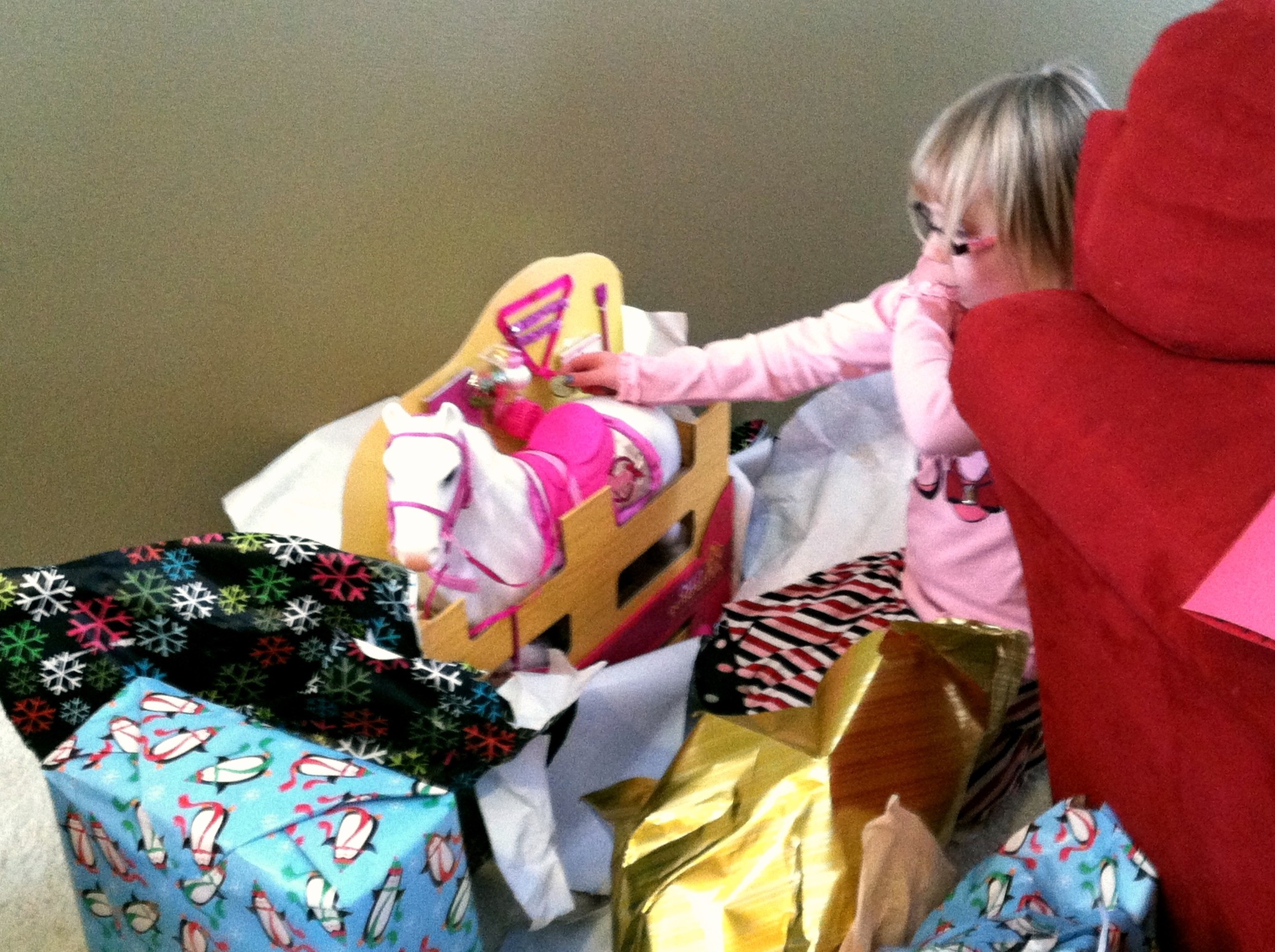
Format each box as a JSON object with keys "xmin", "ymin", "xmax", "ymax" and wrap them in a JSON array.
[{"xmin": 562, "ymin": 65, "xmax": 1110, "ymax": 840}]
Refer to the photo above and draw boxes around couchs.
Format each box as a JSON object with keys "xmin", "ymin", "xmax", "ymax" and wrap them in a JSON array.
[{"xmin": 947, "ymin": 286, "xmax": 1275, "ymax": 952}]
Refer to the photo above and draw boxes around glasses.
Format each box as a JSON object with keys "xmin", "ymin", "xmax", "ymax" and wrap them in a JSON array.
[{"xmin": 909, "ymin": 201, "xmax": 998, "ymax": 263}]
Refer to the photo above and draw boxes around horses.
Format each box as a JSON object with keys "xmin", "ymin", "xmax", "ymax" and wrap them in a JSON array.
[{"xmin": 380, "ymin": 390, "xmax": 695, "ymax": 670}]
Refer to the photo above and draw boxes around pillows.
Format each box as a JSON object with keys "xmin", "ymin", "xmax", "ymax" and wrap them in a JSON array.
[{"xmin": 1044, "ymin": 0, "xmax": 1275, "ymax": 386}]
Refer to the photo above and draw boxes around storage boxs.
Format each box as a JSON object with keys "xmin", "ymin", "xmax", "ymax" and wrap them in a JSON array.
[
  {"xmin": 910, "ymin": 796, "xmax": 1159, "ymax": 952},
  {"xmin": 34, "ymin": 665, "xmax": 486, "ymax": 952}
]
